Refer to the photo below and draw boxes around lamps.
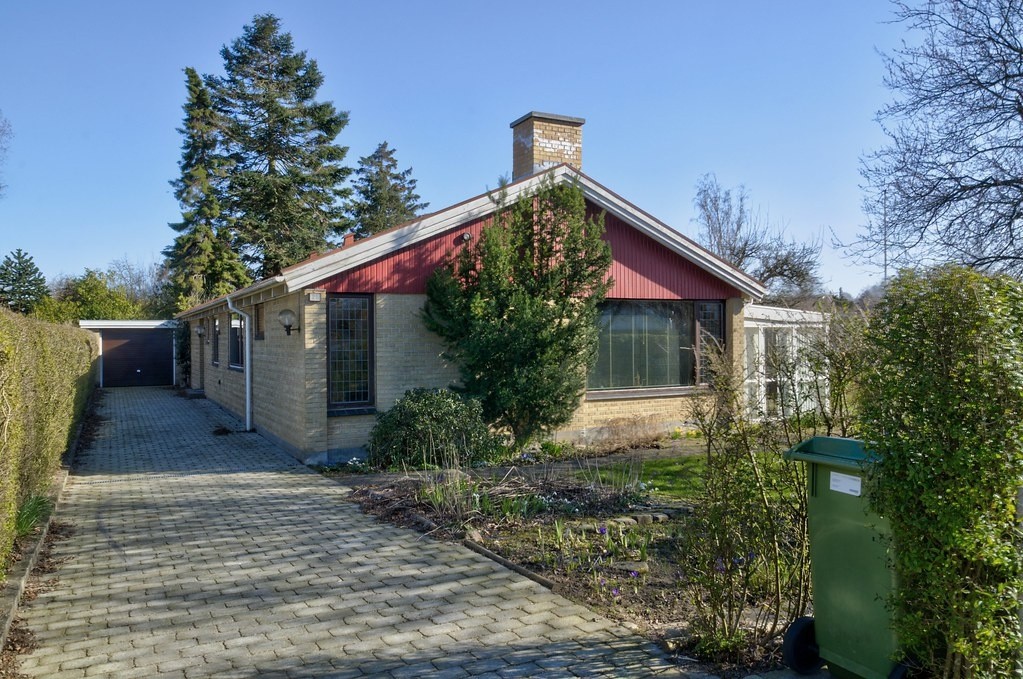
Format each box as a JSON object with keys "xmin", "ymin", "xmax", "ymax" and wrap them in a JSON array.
[
  {"xmin": 195, "ymin": 326, "xmax": 206, "ymax": 337},
  {"xmin": 277, "ymin": 309, "xmax": 300, "ymax": 336}
]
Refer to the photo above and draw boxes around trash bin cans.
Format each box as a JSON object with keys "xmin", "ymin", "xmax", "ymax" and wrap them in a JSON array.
[{"xmin": 782, "ymin": 437, "xmax": 948, "ymax": 679}]
